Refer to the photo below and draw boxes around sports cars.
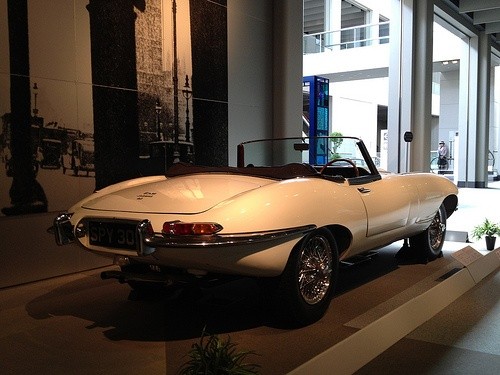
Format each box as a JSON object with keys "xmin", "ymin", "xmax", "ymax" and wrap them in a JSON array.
[{"xmin": 47, "ymin": 136, "xmax": 459, "ymax": 324}]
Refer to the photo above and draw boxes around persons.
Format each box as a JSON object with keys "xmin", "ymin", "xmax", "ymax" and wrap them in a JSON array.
[{"xmin": 437, "ymin": 141, "xmax": 448, "ymax": 173}]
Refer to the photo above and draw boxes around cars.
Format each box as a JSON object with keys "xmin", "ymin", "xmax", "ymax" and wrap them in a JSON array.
[{"xmin": 59, "ymin": 138, "xmax": 96, "ymax": 177}]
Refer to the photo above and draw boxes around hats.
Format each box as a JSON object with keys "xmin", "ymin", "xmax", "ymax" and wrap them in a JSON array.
[{"xmin": 440, "ymin": 141, "xmax": 445, "ymax": 144}]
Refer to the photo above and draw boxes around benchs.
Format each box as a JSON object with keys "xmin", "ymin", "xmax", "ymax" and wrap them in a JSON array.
[{"xmin": 165, "ymin": 162, "xmax": 345, "ymax": 183}]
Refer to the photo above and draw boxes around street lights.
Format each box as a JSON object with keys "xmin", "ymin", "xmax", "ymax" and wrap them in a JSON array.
[
  {"xmin": 31, "ymin": 81, "xmax": 39, "ymax": 118},
  {"xmin": 153, "ymin": 99, "xmax": 163, "ymax": 146},
  {"xmin": 182, "ymin": 73, "xmax": 193, "ymax": 159}
]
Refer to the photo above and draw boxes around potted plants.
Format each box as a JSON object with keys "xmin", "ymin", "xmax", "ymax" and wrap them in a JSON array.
[{"xmin": 470, "ymin": 217, "xmax": 500, "ymax": 250}]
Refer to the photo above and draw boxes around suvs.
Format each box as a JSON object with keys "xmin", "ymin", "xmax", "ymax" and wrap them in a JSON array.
[{"xmin": 39, "ymin": 139, "xmax": 63, "ymax": 169}]
